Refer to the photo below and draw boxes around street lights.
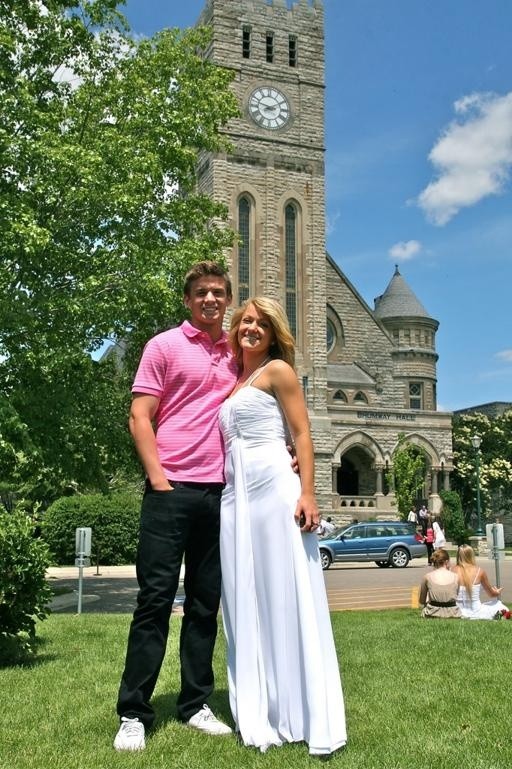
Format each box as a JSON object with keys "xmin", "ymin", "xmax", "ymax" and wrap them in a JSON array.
[{"xmin": 470, "ymin": 435, "xmax": 483, "ymax": 527}]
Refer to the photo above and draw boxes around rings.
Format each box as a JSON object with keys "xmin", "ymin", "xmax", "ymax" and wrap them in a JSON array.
[{"xmin": 313, "ymin": 521, "xmax": 319, "ymax": 524}]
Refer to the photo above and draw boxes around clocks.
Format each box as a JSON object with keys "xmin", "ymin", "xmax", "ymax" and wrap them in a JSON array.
[{"xmin": 243, "ymin": 81, "xmax": 294, "ymax": 135}]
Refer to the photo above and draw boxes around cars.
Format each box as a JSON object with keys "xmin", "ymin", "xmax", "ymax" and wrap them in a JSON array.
[{"xmin": 317, "ymin": 519, "xmax": 428, "ymax": 570}]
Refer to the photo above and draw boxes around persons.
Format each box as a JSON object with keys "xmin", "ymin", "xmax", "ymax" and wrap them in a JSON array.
[
  {"xmin": 217, "ymin": 298, "xmax": 347, "ymax": 754},
  {"xmin": 112, "ymin": 261, "xmax": 299, "ymax": 751},
  {"xmin": 320, "ymin": 505, "xmax": 511, "ymax": 620}
]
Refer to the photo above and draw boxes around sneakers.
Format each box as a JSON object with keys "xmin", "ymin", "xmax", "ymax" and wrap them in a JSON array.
[
  {"xmin": 115, "ymin": 718, "xmax": 146, "ymax": 753},
  {"xmin": 188, "ymin": 708, "xmax": 232, "ymax": 736}
]
